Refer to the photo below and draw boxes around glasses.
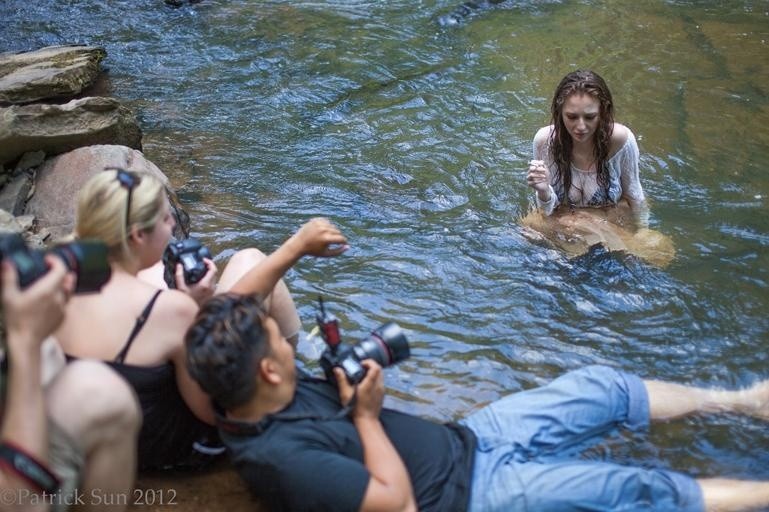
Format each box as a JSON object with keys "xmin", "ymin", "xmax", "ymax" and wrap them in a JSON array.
[{"xmin": 104, "ymin": 165, "xmax": 141, "ymax": 241}]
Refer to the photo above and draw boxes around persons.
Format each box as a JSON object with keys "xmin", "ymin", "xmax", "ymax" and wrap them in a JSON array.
[
  {"xmin": 181, "ymin": 289, "xmax": 769, "ymax": 512},
  {"xmin": 0, "ymin": 253, "xmax": 144, "ymax": 511},
  {"xmin": 51, "ymin": 166, "xmax": 351, "ymax": 482},
  {"xmin": 528, "ymin": 162, "xmax": 539, "ymax": 167},
  {"xmin": 516, "ymin": 68, "xmax": 675, "ymax": 266}
]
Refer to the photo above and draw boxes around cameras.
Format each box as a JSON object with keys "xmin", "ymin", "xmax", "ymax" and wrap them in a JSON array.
[
  {"xmin": 1, "ymin": 232, "xmax": 111, "ymax": 295},
  {"xmin": 162, "ymin": 238, "xmax": 212, "ymax": 289},
  {"xmin": 315, "ymin": 292, "xmax": 410, "ymax": 385}
]
